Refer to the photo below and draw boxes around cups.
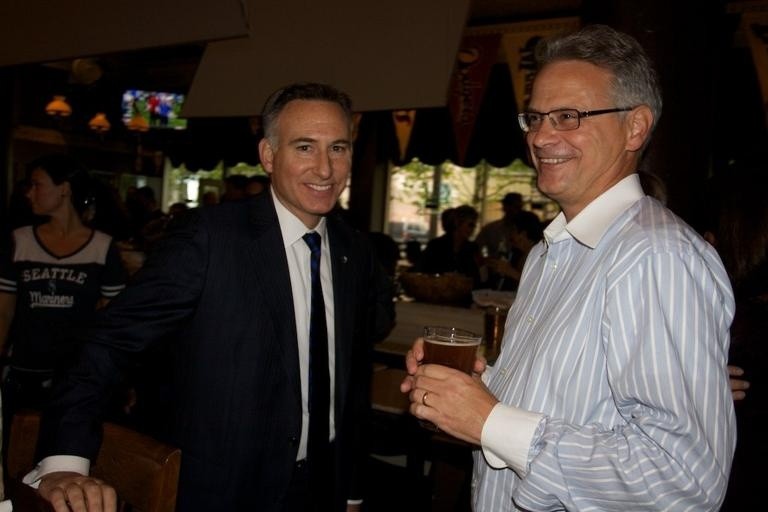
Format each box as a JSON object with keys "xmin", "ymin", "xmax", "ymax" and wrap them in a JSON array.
[
  {"xmin": 484, "ymin": 305, "xmax": 504, "ymax": 340},
  {"xmin": 417, "ymin": 325, "xmax": 482, "ymax": 431}
]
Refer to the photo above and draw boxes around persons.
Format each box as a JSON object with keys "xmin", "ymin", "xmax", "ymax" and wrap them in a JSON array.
[
  {"xmin": 401, "ymin": 25, "xmax": 739, "ymax": 512},
  {"xmin": 641, "ymin": 165, "xmax": 765, "ymax": 484},
  {"xmin": 1, "ymin": 156, "xmax": 129, "ymax": 418},
  {"xmin": 76, "ymin": 173, "xmax": 267, "ymax": 244},
  {"xmin": 410, "ymin": 186, "xmax": 547, "ymax": 305},
  {"xmin": 21, "ymin": 81, "xmax": 375, "ymax": 510}
]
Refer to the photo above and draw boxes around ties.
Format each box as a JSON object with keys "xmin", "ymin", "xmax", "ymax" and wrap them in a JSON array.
[{"xmin": 301, "ymin": 231, "xmax": 331, "ymax": 458}]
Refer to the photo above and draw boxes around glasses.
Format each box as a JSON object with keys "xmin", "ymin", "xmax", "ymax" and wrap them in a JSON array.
[{"xmin": 516, "ymin": 105, "xmax": 639, "ymax": 131}]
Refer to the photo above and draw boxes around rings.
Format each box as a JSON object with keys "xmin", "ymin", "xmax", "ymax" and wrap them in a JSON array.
[{"xmin": 423, "ymin": 393, "xmax": 429, "ymax": 407}]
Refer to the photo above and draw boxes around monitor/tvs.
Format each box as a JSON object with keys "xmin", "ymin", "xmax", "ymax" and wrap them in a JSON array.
[{"xmin": 121, "ymin": 88, "xmax": 189, "ymax": 133}]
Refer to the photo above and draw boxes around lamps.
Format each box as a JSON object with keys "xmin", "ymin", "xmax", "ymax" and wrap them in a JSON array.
[
  {"xmin": 42, "ymin": 70, "xmax": 73, "ymax": 117},
  {"xmin": 87, "ymin": 84, "xmax": 111, "ymax": 133}
]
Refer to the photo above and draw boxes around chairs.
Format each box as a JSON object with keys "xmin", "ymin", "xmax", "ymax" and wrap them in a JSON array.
[{"xmin": 3, "ymin": 405, "xmax": 184, "ymax": 511}]
